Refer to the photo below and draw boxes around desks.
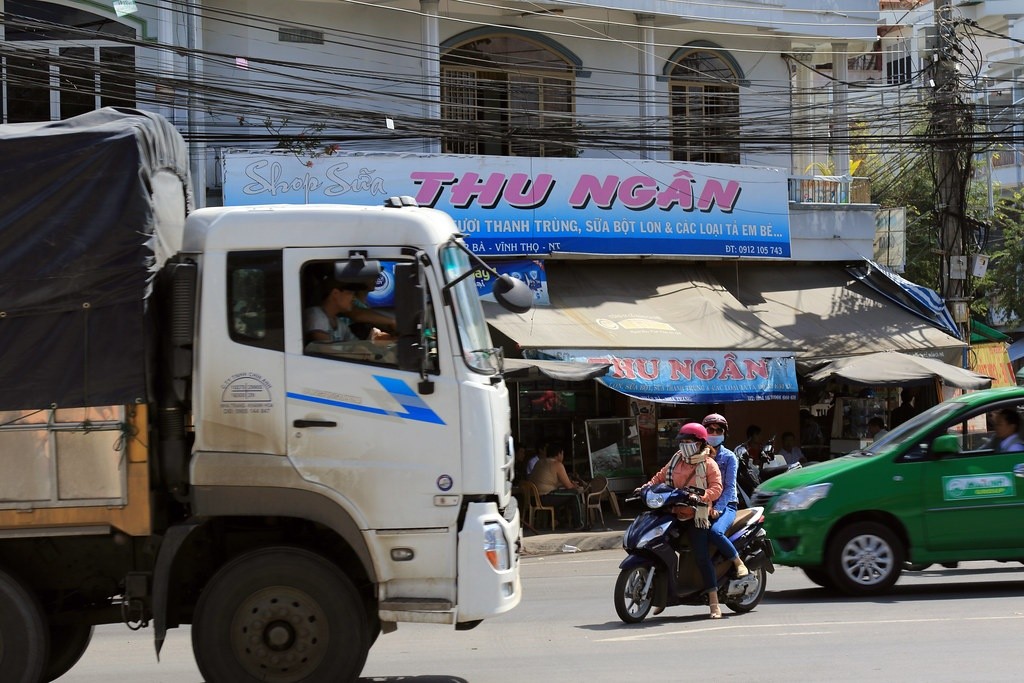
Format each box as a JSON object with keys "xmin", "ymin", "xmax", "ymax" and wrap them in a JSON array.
[{"xmin": 552, "ymin": 487, "xmax": 585, "ymax": 531}]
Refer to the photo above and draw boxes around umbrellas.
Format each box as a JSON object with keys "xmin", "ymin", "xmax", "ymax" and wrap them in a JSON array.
[
  {"xmin": 805, "ymin": 350, "xmax": 998, "ymax": 430},
  {"xmin": 498, "ymin": 357, "xmax": 613, "ymax": 443}
]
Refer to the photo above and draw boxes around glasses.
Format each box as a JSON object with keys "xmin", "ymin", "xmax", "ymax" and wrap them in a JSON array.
[{"xmin": 706, "ymin": 427, "xmax": 725, "ymax": 435}]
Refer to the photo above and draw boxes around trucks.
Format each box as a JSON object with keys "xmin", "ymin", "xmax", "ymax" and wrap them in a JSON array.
[{"xmin": 0, "ymin": 103, "xmax": 534, "ymax": 683}]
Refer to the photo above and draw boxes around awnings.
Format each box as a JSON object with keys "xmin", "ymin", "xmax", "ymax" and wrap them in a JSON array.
[
  {"xmin": 709, "ymin": 265, "xmax": 969, "ymax": 372},
  {"xmin": 470, "ymin": 260, "xmax": 808, "ymax": 356}
]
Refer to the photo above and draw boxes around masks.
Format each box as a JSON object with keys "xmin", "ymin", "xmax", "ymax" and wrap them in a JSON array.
[
  {"xmin": 678, "ymin": 442, "xmax": 701, "ymax": 457},
  {"xmin": 706, "ymin": 435, "xmax": 725, "ymax": 447}
]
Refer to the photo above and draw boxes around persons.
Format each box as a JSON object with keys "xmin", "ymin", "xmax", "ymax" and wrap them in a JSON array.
[
  {"xmin": 887, "ymin": 388, "xmax": 916, "ymax": 431},
  {"xmin": 338, "ymin": 267, "xmax": 398, "ymax": 364},
  {"xmin": 977, "ymin": 409, "xmax": 1024, "ymax": 452},
  {"xmin": 778, "ymin": 431, "xmax": 808, "ymax": 469},
  {"xmin": 801, "ymin": 410, "xmax": 823, "ymax": 461},
  {"xmin": 512, "ymin": 443, "xmax": 525, "ymax": 488},
  {"xmin": 830, "ymin": 383, "xmax": 850, "ymax": 407},
  {"xmin": 868, "ymin": 418, "xmax": 889, "ymax": 443},
  {"xmin": 634, "ymin": 423, "xmax": 722, "ymax": 620},
  {"xmin": 529, "ymin": 444, "xmax": 584, "ymax": 531},
  {"xmin": 745, "ymin": 424, "xmax": 765, "ymax": 461},
  {"xmin": 527, "ymin": 437, "xmax": 553, "ymax": 476},
  {"xmin": 702, "ymin": 413, "xmax": 748, "ymax": 579},
  {"xmin": 302, "ymin": 278, "xmax": 378, "ymax": 363}
]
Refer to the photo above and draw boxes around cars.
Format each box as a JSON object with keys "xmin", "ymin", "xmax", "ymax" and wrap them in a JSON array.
[{"xmin": 751, "ymin": 385, "xmax": 1024, "ymax": 599}]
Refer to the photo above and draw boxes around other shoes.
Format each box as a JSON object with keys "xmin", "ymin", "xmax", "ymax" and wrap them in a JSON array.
[
  {"xmin": 736, "ymin": 563, "xmax": 749, "ymax": 577},
  {"xmin": 653, "ymin": 606, "xmax": 664, "ymax": 615},
  {"xmin": 710, "ymin": 603, "xmax": 722, "ymax": 618}
]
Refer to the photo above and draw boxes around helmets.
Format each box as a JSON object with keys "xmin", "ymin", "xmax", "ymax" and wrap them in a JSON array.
[
  {"xmin": 702, "ymin": 413, "xmax": 728, "ymax": 433},
  {"xmin": 675, "ymin": 422, "xmax": 707, "ymax": 443}
]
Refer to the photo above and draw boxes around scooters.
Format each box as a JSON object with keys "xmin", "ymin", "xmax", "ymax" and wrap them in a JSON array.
[
  {"xmin": 613, "ymin": 482, "xmax": 776, "ymax": 624},
  {"xmin": 733, "ymin": 433, "xmax": 822, "ymax": 507}
]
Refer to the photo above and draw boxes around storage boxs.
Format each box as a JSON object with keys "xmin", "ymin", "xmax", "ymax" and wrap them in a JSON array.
[{"xmin": 830, "ymin": 438, "xmax": 874, "ymax": 454}]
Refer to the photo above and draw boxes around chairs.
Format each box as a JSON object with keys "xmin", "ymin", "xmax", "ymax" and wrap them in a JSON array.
[
  {"xmin": 579, "ymin": 475, "xmax": 609, "ymax": 532},
  {"xmin": 520, "ymin": 480, "xmax": 555, "ymax": 535}
]
